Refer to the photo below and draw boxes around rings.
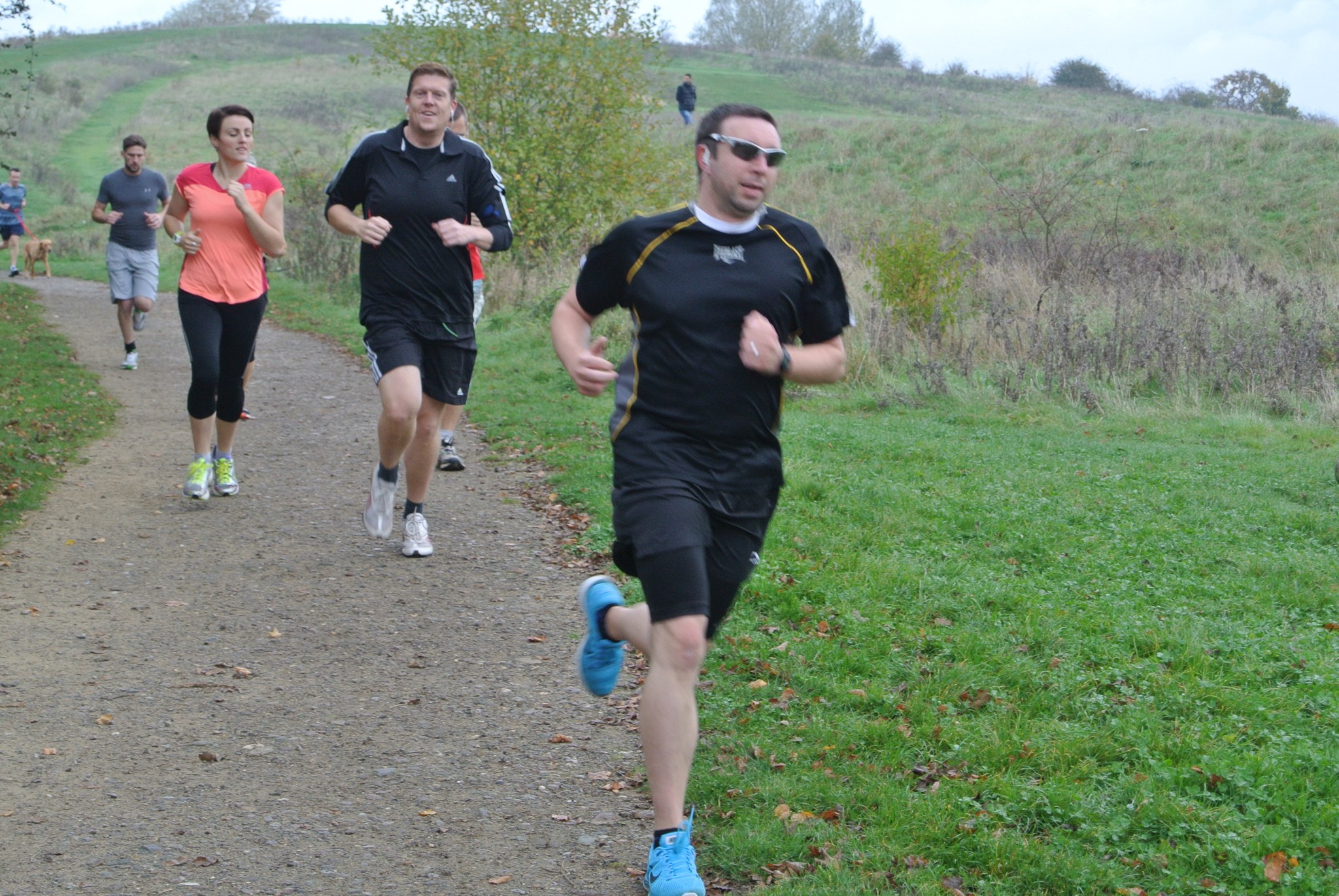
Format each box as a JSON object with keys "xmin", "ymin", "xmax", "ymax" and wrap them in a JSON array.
[{"xmin": 750, "ymin": 342, "xmax": 759, "ymax": 356}]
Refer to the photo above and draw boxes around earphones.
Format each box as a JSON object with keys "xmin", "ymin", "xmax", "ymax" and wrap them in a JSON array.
[
  {"xmin": 450, "ymin": 107, "xmax": 454, "ymax": 116},
  {"xmin": 405, "ymin": 105, "xmax": 409, "ymax": 112},
  {"xmin": 702, "ymin": 149, "xmax": 710, "ymax": 167}
]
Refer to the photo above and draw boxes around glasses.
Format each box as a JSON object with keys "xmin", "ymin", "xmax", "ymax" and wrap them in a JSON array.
[{"xmin": 709, "ymin": 133, "xmax": 788, "ymax": 167}]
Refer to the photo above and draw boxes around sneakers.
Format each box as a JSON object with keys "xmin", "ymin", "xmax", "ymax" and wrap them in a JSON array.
[
  {"xmin": 183, "ymin": 460, "xmax": 213, "ymax": 501},
  {"xmin": 212, "ymin": 444, "xmax": 240, "ymax": 496},
  {"xmin": 644, "ymin": 804, "xmax": 706, "ymax": 896},
  {"xmin": 362, "ymin": 460, "xmax": 400, "ymax": 539},
  {"xmin": 438, "ymin": 435, "xmax": 466, "ymax": 470},
  {"xmin": 400, "ymin": 511, "xmax": 434, "ymax": 556},
  {"xmin": 133, "ymin": 307, "xmax": 146, "ymax": 332},
  {"xmin": 576, "ymin": 576, "xmax": 624, "ymax": 698},
  {"xmin": 121, "ymin": 349, "xmax": 139, "ymax": 370}
]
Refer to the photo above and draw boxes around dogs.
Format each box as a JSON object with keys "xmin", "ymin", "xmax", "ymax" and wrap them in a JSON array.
[{"xmin": 21, "ymin": 237, "xmax": 54, "ymax": 280}]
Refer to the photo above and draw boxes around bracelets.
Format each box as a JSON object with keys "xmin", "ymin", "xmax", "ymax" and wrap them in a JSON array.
[{"xmin": 778, "ymin": 342, "xmax": 792, "ymax": 380}]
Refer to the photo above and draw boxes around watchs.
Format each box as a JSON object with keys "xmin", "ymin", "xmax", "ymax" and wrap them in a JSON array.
[{"xmin": 172, "ymin": 231, "xmax": 186, "ymax": 247}]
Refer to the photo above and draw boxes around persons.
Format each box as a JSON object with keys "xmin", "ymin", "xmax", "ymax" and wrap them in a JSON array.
[
  {"xmin": 166, "ymin": 105, "xmax": 287, "ymax": 501},
  {"xmin": 324, "ymin": 63, "xmax": 514, "ymax": 558},
  {"xmin": 92, "ymin": 133, "xmax": 171, "ymax": 370},
  {"xmin": 435, "ymin": 102, "xmax": 486, "ymax": 472},
  {"xmin": 0, "ymin": 168, "xmax": 27, "ymax": 277},
  {"xmin": 676, "ymin": 73, "xmax": 696, "ymax": 125},
  {"xmin": 551, "ymin": 104, "xmax": 858, "ymax": 896}
]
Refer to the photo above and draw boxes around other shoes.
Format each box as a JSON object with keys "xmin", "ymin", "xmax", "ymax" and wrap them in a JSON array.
[{"xmin": 9, "ymin": 268, "xmax": 19, "ymax": 277}]
